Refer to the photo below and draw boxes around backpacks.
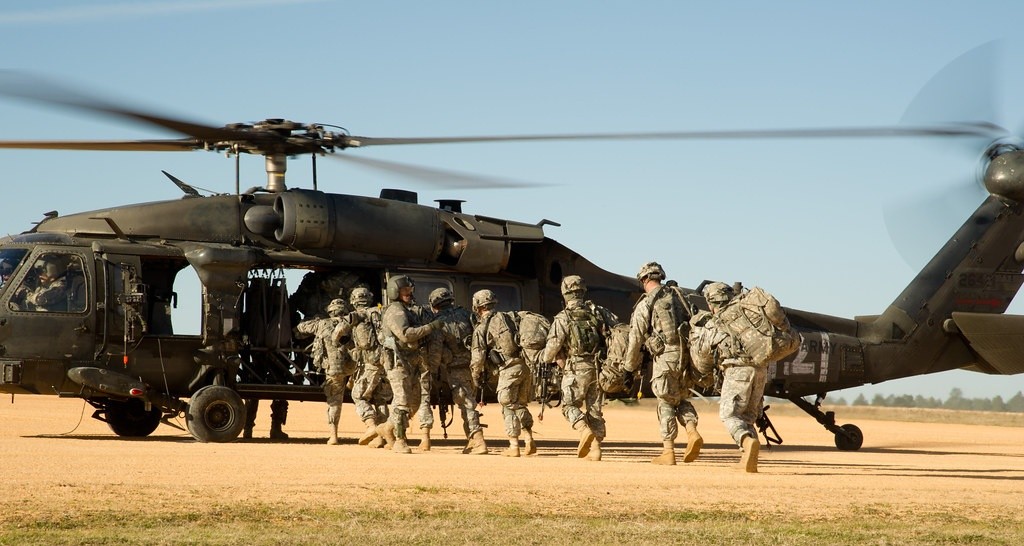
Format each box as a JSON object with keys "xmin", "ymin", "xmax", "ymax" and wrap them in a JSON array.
[
  {"xmin": 320, "ymin": 268, "xmax": 373, "ymax": 309},
  {"xmin": 507, "ymin": 308, "xmax": 550, "ymax": 370},
  {"xmin": 594, "ymin": 307, "xmax": 644, "ymax": 395},
  {"xmin": 716, "ymin": 287, "xmax": 801, "ymax": 368},
  {"xmin": 669, "ymin": 284, "xmax": 717, "ymax": 389}
]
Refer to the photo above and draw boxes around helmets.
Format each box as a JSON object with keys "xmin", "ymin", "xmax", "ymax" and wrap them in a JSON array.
[
  {"xmin": 636, "ymin": 262, "xmax": 666, "ymax": 281},
  {"xmin": 0, "ymin": 259, "xmax": 20, "ymax": 274},
  {"xmin": 561, "ymin": 275, "xmax": 587, "ymax": 294},
  {"xmin": 703, "ymin": 281, "xmax": 734, "ymax": 302},
  {"xmin": 325, "ymin": 275, "xmax": 499, "ymax": 318},
  {"xmin": 33, "ymin": 250, "xmax": 68, "ymax": 280}
]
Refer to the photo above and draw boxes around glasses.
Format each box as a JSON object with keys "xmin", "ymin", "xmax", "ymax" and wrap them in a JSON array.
[{"xmin": 399, "ymin": 286, "xmax": 415, "ymax": 295}]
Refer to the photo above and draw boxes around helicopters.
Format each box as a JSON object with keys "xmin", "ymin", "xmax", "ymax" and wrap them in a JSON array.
[{"xmin": 0, "ymin": 70, "xmax": 1023, "ymax": 450}]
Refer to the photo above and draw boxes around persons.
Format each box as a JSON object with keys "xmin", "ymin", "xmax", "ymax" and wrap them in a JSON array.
[
  {"xmin": 623, "ymin": 262, "xmax": 703, "ymax": 466},
  {"xmin": 690, "ymin": 282, "xmax": 765, "ymax": 474},
  {"xmin": 543, "ymin": 275, "xmax": 620, "ymax": 462},
  {"xmin": 297, "ymin": 287, "xmax": 487, "ymax": 456},
  {"xmin": 470, "ymin": 289, "xmax": 536, "ymax": 456},
  {"xmin": 28, "ymin": 258, "xmax": 68, "ymax": 312},
  {"xmin": 375, "ymin": 274, "xmax": 445, "ymax": 454},
  {"xmin": 241, "ymin": 285, "xmax": 289, "ymax": 439}
]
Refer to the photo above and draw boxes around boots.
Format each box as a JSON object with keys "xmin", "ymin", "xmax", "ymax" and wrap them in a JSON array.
[
  {"xmin": 652, "ymin": 439, "xmax": 677, "ymax": 466},
  {"xmin": 242, "ymin": 426, "xmax": 253, "ymax": 438},
  {"xmin": 682, "ymin": 421, "xmax": 703, "ymax": 463},
  {"xmin": 524, "ymin": 430, "xmax": 536, "ymax": 455},
  {"xmin": 501, "ymin": 436, "xmax": 520, "ymax": 457},
  {"xmin": 740, "ymin": 433, "xmax": 759, "ymax": 472},
  {"xmin": 270, "ymin": 417, "xmax": 288, "ymax": 440},
  {"xmin": 417, "ymin": 428, "xmax": 431, "ymax": 451},
  {"xmin": 346, "ymin": 375, "xmax": 354, "ymax": 390},
  {"xmin": 327, "ymin": 425, "xmax": 339, "ymax": 445},
  {"xmin": 358, "ymin": 419, "xmax": 411, "ymax": 453},
  {"xmin": 462, "ymin": 430, "xmax": 489, "ymax": 454},
  {"xmin": 586, "ymin": 437, "xmax": 601, "ymax": 462},
  {"xmin": 574, "ymin": 420, "xmax": 594, "ymax": 458}
]
